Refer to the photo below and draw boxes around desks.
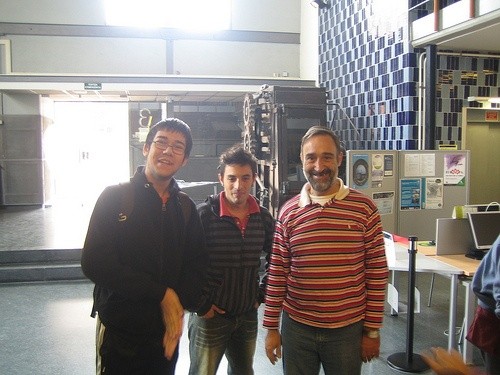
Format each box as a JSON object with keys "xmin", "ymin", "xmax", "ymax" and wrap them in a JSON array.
[
  {"xmin": 383, "ymin": 231, "xmax": 485, "ymax": 367},
  {"xmin": 177, "ymin": 179, "xmax": 221, "ymax": 195}
]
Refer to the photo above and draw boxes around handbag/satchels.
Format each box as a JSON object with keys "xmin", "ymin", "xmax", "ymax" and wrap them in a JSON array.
[{"xmin": 465, "ymin": 305, "xmax": 500, "ymax": 356}]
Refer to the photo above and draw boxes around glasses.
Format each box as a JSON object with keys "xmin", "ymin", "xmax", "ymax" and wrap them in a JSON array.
[{"xmin": 151, "ymin": 139, "xmax": 187, "ymax": 155}]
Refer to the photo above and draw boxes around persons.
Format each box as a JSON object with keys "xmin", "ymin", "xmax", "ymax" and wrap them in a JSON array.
[
  {"xmin": 421, "ymin": 232, "xmax": 499, "ymax": 375},
  {"xmin": 81, "ymin": 118, "xmax": 204, "ymax": 375},
  {"xmin": 262, "ymin": 126, "xmax": 388, "ymax": 375},
  {"xmin": 187, "ymin": 146, "xmax": 276, "ymax": 375}
]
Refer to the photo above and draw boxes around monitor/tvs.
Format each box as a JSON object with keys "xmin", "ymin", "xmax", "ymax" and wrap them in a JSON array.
[{"xmin": 467, "ymin": 211, "xmax": 500, "ymax": 249}]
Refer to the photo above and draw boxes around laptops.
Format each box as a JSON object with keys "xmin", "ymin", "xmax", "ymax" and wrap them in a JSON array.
[{"xmin": 436, "ymin": 218, "xmax": 473, "ymax": 255}]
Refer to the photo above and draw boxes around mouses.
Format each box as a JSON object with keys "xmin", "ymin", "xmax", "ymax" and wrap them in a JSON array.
[{"xmin": 429, "ymin": 240, "xmax": 435, "ymax": 245}]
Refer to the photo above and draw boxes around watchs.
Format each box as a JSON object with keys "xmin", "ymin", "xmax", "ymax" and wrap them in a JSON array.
[{"xmin": 363, "ymin": 330, "xmax": 380, "ymax": 340}]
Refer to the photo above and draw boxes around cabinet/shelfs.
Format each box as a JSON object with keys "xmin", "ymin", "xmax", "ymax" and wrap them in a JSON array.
[
  {"xmin": 0, "ymin": 92, "xmax": 44, "ymax": 208},
  {"xmin": 243, "ymin": 86, "xmax": 327, "ymax": 219}
]
